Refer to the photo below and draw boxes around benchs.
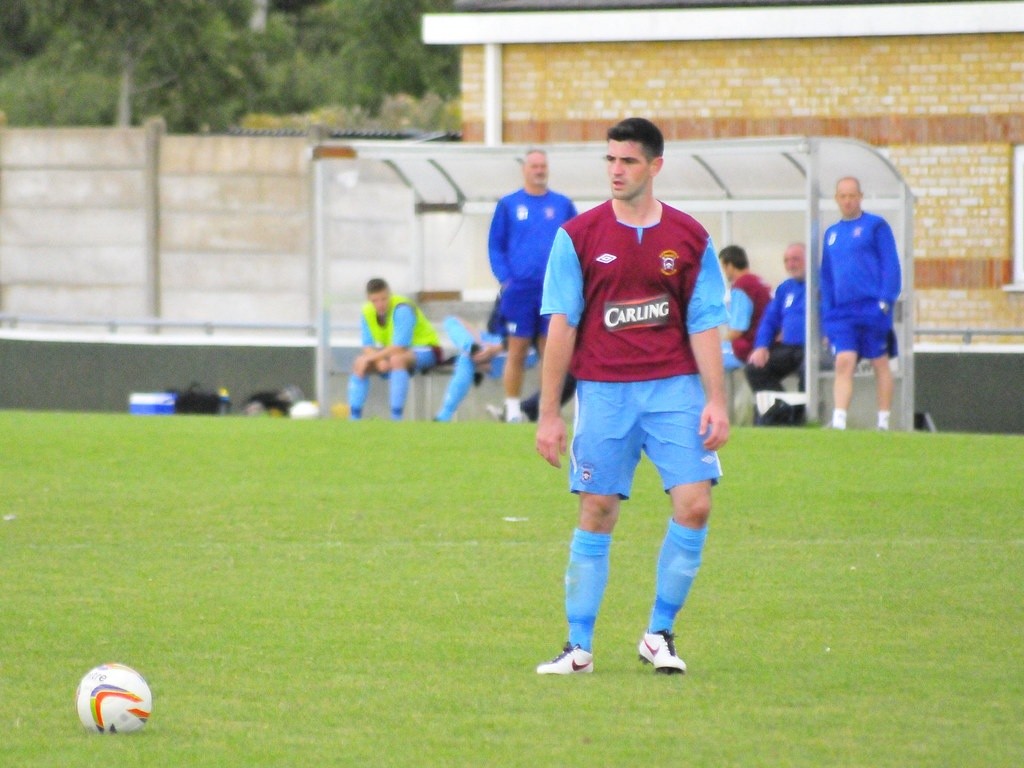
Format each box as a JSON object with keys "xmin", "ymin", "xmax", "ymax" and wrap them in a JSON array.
[{"xmin": 413, "ymin": 364, "xmax": 453, "ymax": 422}]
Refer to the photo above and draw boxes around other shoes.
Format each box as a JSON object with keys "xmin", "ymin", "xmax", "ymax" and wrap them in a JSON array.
[{"xmin": 487, "ymin": 404, "xmax": 537, "ymax": 424}]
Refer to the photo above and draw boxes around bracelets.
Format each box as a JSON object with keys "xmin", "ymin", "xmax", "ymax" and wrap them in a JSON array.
[{"xmin": 880, "ymin": 303, "xmax": 886, "ymax": 309}]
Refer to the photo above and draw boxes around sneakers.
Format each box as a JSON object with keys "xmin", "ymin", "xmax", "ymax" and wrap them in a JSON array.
[
  {"xmin": 536, "ymin": 642, "xmax": 594, "ymax": 673},
  {"xmin": 638, "ymin": 629, "xmax": 686, "ymax": 674}
]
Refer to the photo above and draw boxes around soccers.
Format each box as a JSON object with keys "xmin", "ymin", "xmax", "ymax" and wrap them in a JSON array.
[{"xmin": 77, "ymin": 660, "xmax": 152, "ymax": 736}]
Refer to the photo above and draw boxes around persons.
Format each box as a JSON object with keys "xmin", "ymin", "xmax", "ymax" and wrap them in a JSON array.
[
  {"xmin": 486, "ymin": 373, "xmax": 576, "ymax": 422},
  {"xmin": 719, "ymin": 244, "xmax": 772, "ymax": 370},
  {"xmin": 433, "ymin": 317, "xmax": 540, "ymax": 422},
  {"xmin": 488, "ymin": 149, "xmax": 578, "ymax": 423},
  {"xmin": 743, "ymin": 243, "xmax": 834, "ymax": 426},
  {"xmin": 819, "ymin": 176, "xmax": 901, "ymax": 430},
  {"xmin": 348, "ymin": 278, "xmax": 442, "ymax": 422},
  {"xmin": 537, "ymin": 118, "xmax": 730, "ymax": 674}
]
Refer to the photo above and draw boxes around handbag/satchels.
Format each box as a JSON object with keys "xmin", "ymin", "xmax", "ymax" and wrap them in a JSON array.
[{"xmin": 165, "ymin": 381, "xmax": 221, "ymax": 415}]
